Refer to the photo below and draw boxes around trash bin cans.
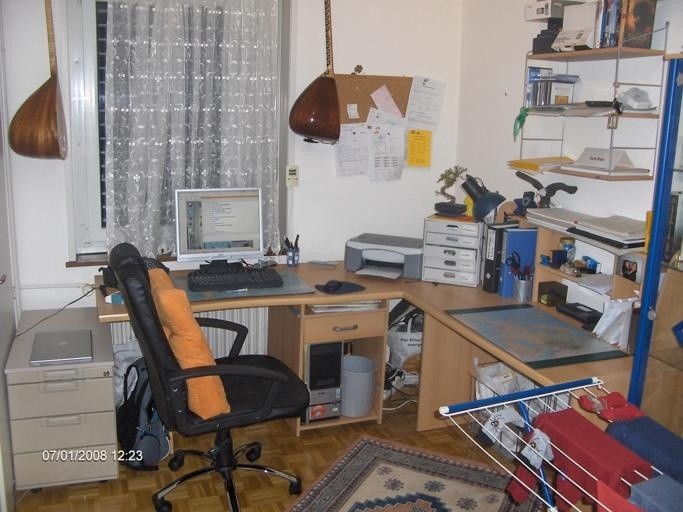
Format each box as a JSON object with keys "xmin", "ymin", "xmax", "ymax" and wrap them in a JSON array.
[{"xmin": 341, "ymin": 355, "xmax": 377, "ymax": 417}]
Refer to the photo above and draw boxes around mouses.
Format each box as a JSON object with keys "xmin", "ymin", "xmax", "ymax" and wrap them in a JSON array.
[{"xmin": 325, "ymin": 280, "xmax": 341, "ymax": 291}]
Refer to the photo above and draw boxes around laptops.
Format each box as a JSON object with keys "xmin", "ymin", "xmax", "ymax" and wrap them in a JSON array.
[{"xmin": 29, "ymin": 329, "xmax": 92, "ymax": 364}]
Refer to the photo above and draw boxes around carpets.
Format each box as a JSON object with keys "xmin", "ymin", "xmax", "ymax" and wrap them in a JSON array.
[{"xmin": 287, "ymin": 429, "xmax": 539, "ymax": 512}]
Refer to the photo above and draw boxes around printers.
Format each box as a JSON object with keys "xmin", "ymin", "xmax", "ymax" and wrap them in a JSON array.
[{"xmin": 344, "ymin": 233, "xmax": 423, "ymax": 281}]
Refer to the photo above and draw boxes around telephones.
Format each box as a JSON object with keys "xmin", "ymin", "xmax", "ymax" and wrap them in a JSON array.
[{"xmin": 143, "ymin": 257, "xmax": 169, "ymax": 275}]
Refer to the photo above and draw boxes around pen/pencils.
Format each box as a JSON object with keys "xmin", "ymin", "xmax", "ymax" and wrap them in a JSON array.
[
  {"xmin": 510, "ymin": 264, "xmax": 535, "ymax": 280},
  {"xmin": 284, "ymin": 234, "xmax": 299, "ymax": 251}
]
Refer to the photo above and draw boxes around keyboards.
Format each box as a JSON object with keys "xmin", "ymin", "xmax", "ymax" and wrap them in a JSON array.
[{"xmin": 188, "ymin": 267, "xmax": 283, "ymax": 291}]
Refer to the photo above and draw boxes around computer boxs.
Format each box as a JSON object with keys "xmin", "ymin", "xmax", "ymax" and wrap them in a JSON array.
[{"xmin": 302, "ymin": 341, "xmax": 344, "ymax": 424}]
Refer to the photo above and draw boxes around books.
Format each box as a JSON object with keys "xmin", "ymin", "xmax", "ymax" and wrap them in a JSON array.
[
  {"xmin": 590, "ymin": 0, "xmax": 658, "ymax": 51},
  {"xmin": 565, "ymin": 214, "xmax": 648, "ymax": 257},
  {"xmin": 308, "ymin": 299, "xmax": 382, "ymax": 317}
]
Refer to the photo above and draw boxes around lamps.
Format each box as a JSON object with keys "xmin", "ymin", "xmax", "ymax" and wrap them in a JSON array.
[{"xmin": 463, "ymin": 173, "xmax": 505, "ymax": 223}]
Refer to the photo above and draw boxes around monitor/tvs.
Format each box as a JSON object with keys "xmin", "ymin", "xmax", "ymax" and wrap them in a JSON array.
[{"xmin": 175, "ymin": 188, "xmax": 263, "ymax": 268}]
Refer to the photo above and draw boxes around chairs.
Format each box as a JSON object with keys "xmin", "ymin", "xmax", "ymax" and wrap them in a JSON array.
[{"xmin": 109, "ymin": 239, "xmax": 310, "ymax": 511}]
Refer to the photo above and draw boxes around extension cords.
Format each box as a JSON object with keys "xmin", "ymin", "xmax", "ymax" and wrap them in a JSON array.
[{"xmin": 383, "ymin": 380, "xmax": 403, "ymax": 400}]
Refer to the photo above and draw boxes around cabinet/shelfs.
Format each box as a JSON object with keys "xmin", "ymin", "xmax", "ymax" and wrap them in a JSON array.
[
  {"xmin": 531, "ymin": 223, "xmax": 646, "ymax": 335},
  {"xmin": 421, "ymin": 213, "xmax": 486, "ymax": 287},
  {"xmin": 627, "ymin": 53, "xmax": 683, "ymax": 438},
  {"xmin": 521, "ymin": 15, "xmax": 669, "ymax": 181},
  {"xmin": 4, "ymin": 307, "xmax": 117, "ymax": 495}
]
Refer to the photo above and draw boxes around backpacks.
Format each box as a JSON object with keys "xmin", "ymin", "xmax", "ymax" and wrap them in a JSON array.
[{"xmin": 116, "ymin": 357, "xmax": 171, "ymax": 471}]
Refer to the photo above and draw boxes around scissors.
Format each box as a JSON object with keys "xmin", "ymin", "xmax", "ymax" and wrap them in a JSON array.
[{"xmin": 505, "ymin": 252, "xmax": 520, "ymax": 268}]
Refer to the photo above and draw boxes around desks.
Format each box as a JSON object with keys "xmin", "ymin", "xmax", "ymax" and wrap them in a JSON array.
[{"xmin": 91, "ymin": 262, "xmax": 681, "ymax": 439}]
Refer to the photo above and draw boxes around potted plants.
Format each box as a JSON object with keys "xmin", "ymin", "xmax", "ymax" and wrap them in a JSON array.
[{"xmin": 430, "ymin": 164, "xmax": 468, "ymax": 217}]
